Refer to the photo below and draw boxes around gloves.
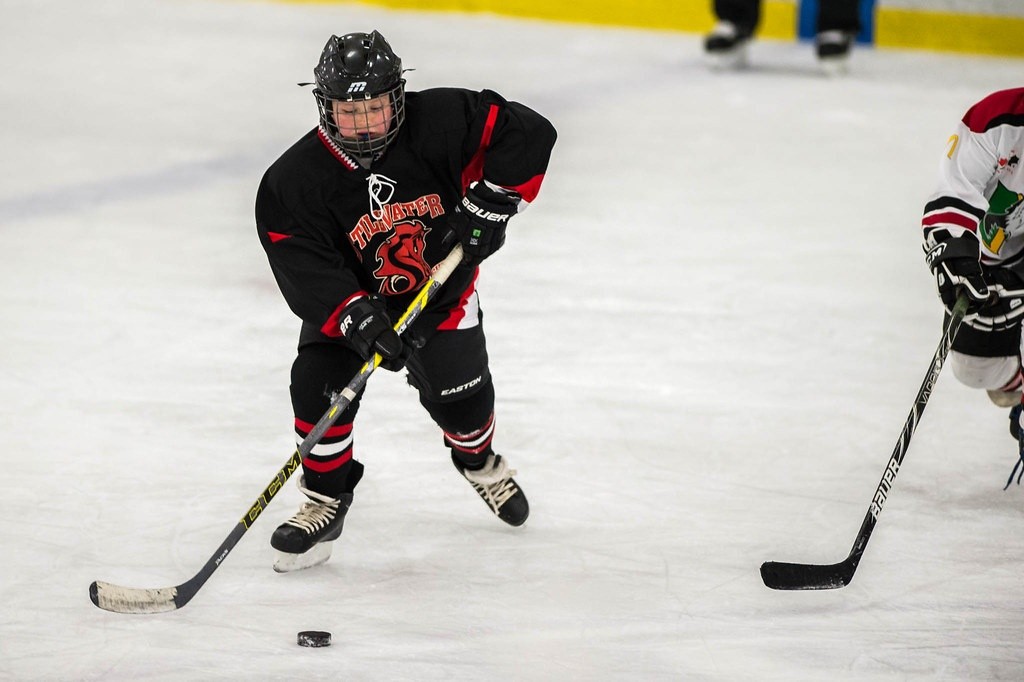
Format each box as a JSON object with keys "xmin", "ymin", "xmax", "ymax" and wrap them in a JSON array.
[
  {"xmin": 440, "ymin": 179, "xmax": 522, "ymax": 270},
  {"xmin": 337, "ymin": 294, "xmax": 414, "ymax": 372},
  {"xmin": 922, "ymin": 223, "xmax": 999, "ymax": 323}
]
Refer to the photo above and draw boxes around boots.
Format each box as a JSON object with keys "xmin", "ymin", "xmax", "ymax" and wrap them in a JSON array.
[
  {"xmin": 442, "ymin": 434, "xmax": 530, "ymax": 527},
  {"xmin": 270, "ymin": 458, "xmax": 365, "ymax": 575}
]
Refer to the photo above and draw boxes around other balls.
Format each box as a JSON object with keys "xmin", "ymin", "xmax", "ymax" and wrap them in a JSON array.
[{"xmin": 294, "ymin": 630, "xmax": 333, "ymax": 649}]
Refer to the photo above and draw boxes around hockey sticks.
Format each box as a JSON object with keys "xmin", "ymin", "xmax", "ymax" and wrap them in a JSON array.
[
  {"xmin": 88, "ymin": 244, "xmax": 464, "ymax": 617},
  {"xmin": 760, "ymin": 183, "xmax": 1014, "ymax": 594}
]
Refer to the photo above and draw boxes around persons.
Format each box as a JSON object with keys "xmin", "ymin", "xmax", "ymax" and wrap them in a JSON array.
[
  {"xmin": 700, "ymin": 0, "xmax": 864, "ymax": 73},
  {"xmin": 254, "ymin": 29, "xmax": 559, "ymax": 574},
  {"xmin": 922, "ymin": 86, "xmax": 1024, "ymax": 467}
]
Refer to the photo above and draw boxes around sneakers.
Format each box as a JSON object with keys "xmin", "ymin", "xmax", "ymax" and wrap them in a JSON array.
[
  {"xmin": 810, "ymin": 0, "xmax": 860, "ymax": 75},
  {"xmin": 700, "ymin": 0, "xmax": 762, "ymax": 70}
]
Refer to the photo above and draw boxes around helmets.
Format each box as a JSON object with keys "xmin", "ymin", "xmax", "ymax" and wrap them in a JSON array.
[{"xmin": 297, "ymin": 30, "xmax": 415, "ymax": 159}]
